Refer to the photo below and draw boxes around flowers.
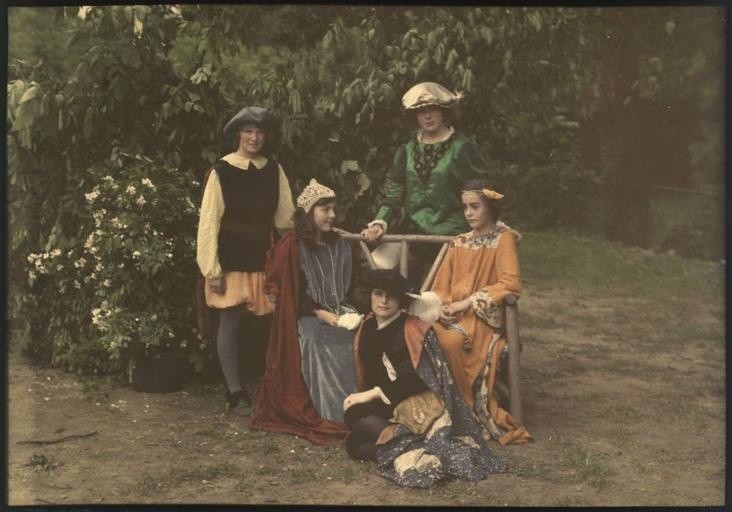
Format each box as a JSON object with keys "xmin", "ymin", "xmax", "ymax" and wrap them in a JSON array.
[{"xmin": 27, "ymin": 165, "xmax": 211, "ymax": 359}]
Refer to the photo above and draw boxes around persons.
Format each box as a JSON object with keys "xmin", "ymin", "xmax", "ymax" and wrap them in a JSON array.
[
  {"xmin": 341, "ymin": 269, "xmax": 510, "ymax": 488},
  {"xmin": 261, "ymin": 177, "xmax": 364, "ymax": 445},
  {"xmin": 429, "ymin": 181, "xmax": 529, "ymax": 445},
  {"xmin": 360, "ymin": 80, "xmax": 487, "ymax": 272},
  {"xmin": 195, "ymin": 106, "xmax": 297, "ymax": 416}
]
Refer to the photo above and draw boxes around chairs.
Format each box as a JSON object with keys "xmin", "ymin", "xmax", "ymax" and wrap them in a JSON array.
[{"xmin": 339, "ymin": 233, "xmax": 523, "ymax": 426}]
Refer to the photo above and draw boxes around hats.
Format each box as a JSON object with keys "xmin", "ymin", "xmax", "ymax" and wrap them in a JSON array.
[
  {"xmin": 295, "ymin": 178, "xmax": 338, "ymax": 213},
  {"xmin": 361, "ymin": 268, "xmax": 412, "ymax": 297},
  {"xmin": 400, "ymin": 81, "xmax": 458, "ymax": 111},
  {"xmin": 221, "ymin": 104, "xmax": 279, "ymax": 141}
]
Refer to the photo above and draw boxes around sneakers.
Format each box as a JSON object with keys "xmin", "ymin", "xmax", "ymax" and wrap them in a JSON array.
[{"xmin": 229, "ymin": 382, "xmax": 251, "ymax": 417}]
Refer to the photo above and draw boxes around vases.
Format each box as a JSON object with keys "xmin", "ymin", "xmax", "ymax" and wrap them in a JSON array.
[{"xmin": 126, "ymin": 352, "xmax": 184, "ymax": 393}]
